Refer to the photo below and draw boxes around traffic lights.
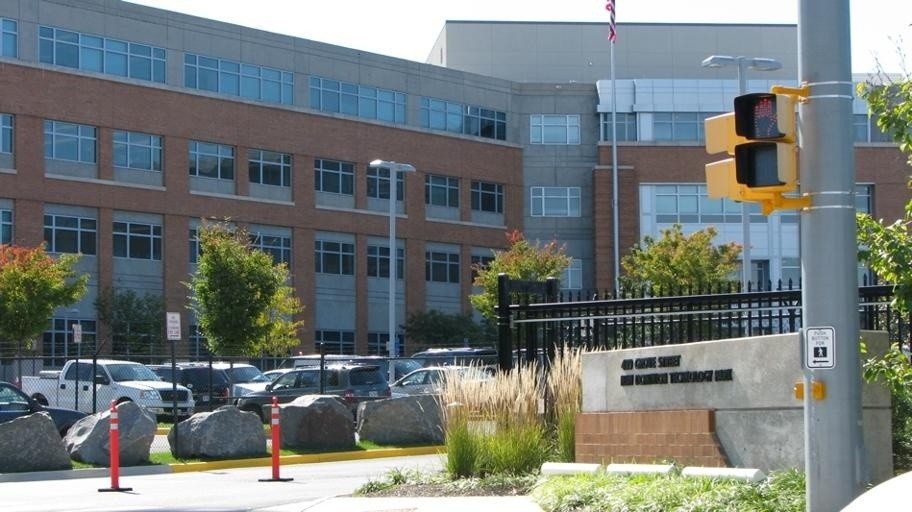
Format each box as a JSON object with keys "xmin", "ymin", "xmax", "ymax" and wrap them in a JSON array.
[{"xmin": 702, "ymin": 91, "xmax": 797, "ymax": 203}]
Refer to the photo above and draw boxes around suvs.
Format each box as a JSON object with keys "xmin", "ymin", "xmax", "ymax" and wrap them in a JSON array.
[{"xmin": 146, "ymin": 362, "xmax": 391, "ymax": 429}]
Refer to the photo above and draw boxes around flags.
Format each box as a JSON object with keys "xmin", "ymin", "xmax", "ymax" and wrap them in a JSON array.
[{"xmin": 607, "ymin": 1, "xmax": 617, "ymax": 45}]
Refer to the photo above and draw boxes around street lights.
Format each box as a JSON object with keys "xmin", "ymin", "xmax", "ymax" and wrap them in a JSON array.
[
  {"xmin": 368, "ymin": 159, "xmax": 415, "ymax": 382},
  {"xmin": 703, "ymin": 54, "xmax": 782, "ymax": 335}
]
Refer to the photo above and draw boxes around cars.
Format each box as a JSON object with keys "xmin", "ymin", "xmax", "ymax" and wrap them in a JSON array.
[
  {"xmin": 390, "ymin": 366, "xmax": 494, "ymax": 395},
  {"xmin": 1, "ymin": 381, "xmax": 85, "ymax": 436}
]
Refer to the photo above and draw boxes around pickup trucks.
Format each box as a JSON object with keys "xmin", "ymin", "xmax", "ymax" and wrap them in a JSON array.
[{"xmin": 16, "ymin": 359, "xmax": 194, "ymax": 423}]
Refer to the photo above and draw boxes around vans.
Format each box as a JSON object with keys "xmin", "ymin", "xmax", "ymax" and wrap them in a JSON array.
[
  {"xmin": 293, "ymin": 354, "xmax": 421, "ymax": 385},
  {"xmin": 410, "ymin": 347, "xmax": 498, "ymax": 376}
]
